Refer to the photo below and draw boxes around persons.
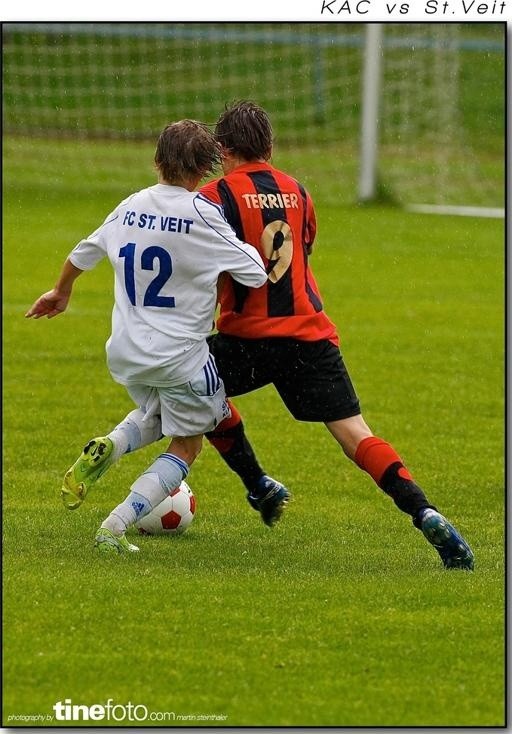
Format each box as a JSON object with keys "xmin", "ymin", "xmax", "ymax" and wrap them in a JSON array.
[
  {"xmin": 24, "ymin": 119, "xmax": 268, "ymax": 552},
  {"xmin": 199, "ymin": 96, "xmax": 477, "ymax": 571}
]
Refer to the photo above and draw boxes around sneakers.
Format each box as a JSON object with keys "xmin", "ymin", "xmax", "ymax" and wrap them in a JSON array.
[
  {"xmin": 420, "ymin": 507, "xmax": 474, "ymax": 570},
  {"xmin": 93, "ymin": 526, "xmax": 140, "ymax": 553},
  {"xmin": 60, "ymin": 436, "xmax": 113, "ymax": 510},
  {"xmin": 247, "ymin": 474, "xmax": 290, "ymax": 527}
]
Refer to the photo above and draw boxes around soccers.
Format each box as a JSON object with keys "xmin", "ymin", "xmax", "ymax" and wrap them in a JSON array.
[{"xmin": 134, "ymin": 480, "xmax": 196, "ymax": 536}]
